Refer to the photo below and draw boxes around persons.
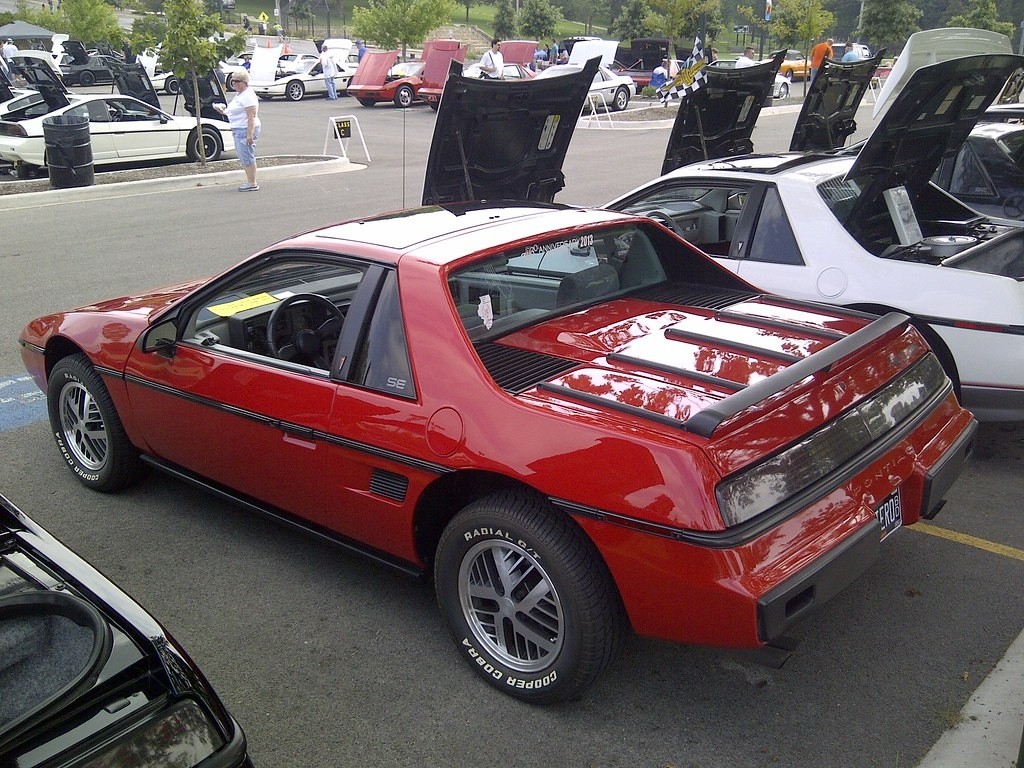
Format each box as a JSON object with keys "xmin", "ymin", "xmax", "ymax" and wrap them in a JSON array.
[
  {"xmin": 321, "ymin": 46, "xmax": 339, "ymax": 100},
  {"xmin": 841, "ymin": 42, "xmax": 858, "ymax": 62},
  {"xmin": 243, "ymin": 16, "xmax": 249, "ymax": 29},
  {"xmin": 735, "ymin": 47, "xmax": 754, "ymax": 69},
  {"xmin": 214, "ymin": 66, "xmax": 260, "ymax": 191},
  {"xmin": 42, "ymin": 0, "xmax": 61, "ymax": 14},
  {"xmin": 0, "ymin": 38, "xmax": 22, "ymax": 81},
  {"xmin": 353, "ymin": 40, "xmax": 366, "ymax": 64},
  {"xmin": 810, "ymin": 39, "xmax": 833, "ymax": 84},
  {"xmin": 711, "ymin": 48, "xmax": 719, "ymax": 61},
  {"xmin": 649, "ymin": 60, "xmax": 671, "ymax": 89},
  {"xmin": 479, "ymin": 39, "xmax": 505, "ymax": 80},
  {"xmin": 242, "ymin": 58, "xmax": 251, "ymax": 72},
  {"xmin": 540, "ymin": 39, "xmax": 558, "ymax": 65}
]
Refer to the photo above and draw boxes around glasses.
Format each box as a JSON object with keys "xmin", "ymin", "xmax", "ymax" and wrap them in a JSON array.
[{"xmin": 231, "ymin": 79, "xmax": 244, "ymax": 84}]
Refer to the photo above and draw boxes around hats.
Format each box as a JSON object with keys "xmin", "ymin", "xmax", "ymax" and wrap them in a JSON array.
[
  {"xmin": 6, "ymin": 38, "xmax": 14, "ymax": 43},
  {"xmin": 844, "ymin": 42, "xmax": 854, "ymax": 48},
  {"xmin": 355, "ymin": 39, "xmax": 361, "ymax": 45}
]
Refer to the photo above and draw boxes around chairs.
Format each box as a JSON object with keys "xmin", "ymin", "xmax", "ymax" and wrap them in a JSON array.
[{"xmin": 556, "ymin": 264, "xmax": 621, "ymax": 311}]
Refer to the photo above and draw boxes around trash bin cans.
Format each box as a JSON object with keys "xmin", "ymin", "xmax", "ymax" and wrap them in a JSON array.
[
  {"xmin": 43, "ymin": 115, "xmax": 95, "ymax": 188},
  {"xmin": 259, "ymin": 22, "xmax": 266, "ymax": 35},
  {"xmin": 763, "ymin": 78, "xmax": 775, "ymax": 107},
  {"xmin": 314, "ymin": 40, "xmax": 324, "ymax": 53}
]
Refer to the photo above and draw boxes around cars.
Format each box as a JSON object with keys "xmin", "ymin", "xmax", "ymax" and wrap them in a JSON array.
[
  {"xmin": 418, "ymin": 54, "xmax": 1024, "ymax": 430},
  {"xmin": 1, "ymin": 24, "xmax": 1024, "ymax": 229},
  {"xmin": 0, "ymin": 489, "xmax": 255, "ymax": 768},
  {"xmin": 13, "ymin": 52, "xmax": 984, "ymax": 710}
]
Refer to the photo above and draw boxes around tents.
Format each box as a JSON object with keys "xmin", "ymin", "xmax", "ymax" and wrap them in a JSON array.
[{"xmin": 0, "ymin": 20, "xmax": 56, "ymax": 39}]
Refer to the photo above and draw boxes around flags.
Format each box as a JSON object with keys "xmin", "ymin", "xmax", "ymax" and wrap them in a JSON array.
[{"xmin": 656, "ymin": 33, "xmax": 708, "ymax": 103}]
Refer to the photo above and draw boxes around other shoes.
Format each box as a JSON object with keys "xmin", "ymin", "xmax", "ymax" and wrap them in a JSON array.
[
  {"xmin": 238, "ymin": 183, "xmax": 259, "ymax": 191},
  {"xmin": 336, "ymin": 97, "xmax": 338, "ymax": 100},
  {"xmin": 326, "ymin": 97, "xmax": 336, "ymax": 100}
]
